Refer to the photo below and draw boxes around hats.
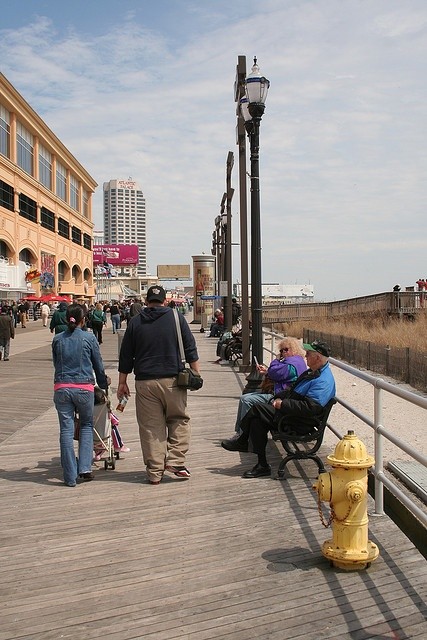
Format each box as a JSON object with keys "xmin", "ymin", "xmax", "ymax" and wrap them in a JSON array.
[
  {"xmin": 303, "ymin": 339, "xmax": 331, "ymax": 357},
  {"xmin": 60, "ymin": 303, "xmax": 67, "ymax": 310},
  {"xmin": 147, "ymin": 286, "xmax": 166, "ymax": 303}
]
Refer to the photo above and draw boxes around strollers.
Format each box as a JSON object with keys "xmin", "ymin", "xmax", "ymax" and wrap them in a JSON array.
[{"xmin": 93, "ymin": 374, "xmax": 119, "ymax": 470}]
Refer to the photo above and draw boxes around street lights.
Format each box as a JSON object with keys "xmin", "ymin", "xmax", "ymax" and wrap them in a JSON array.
[
  {"xmin": 240, "ymin": 55, "xmax": 269, "ymax": 395},
  {"xmin": 221, "ymin": 205, "xmax": 226, "ymax": 334}
]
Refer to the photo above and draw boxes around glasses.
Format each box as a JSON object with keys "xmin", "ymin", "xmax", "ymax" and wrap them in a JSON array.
[{"xmin": 279, "ymin": 347, "xmax": 291, "ymax": 353}]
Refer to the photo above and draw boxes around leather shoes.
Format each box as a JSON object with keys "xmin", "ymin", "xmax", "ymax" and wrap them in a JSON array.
[
  {"xmin": 221, "ymin": 439, "xmax": 248, "ymax": 452},
  {"xmin": 242, "ymin": 463, "xmax": 272, "ymax": 478},
  {"xmin": 4, "ymin": 359, "xmax": 9, "ymax": 361}
]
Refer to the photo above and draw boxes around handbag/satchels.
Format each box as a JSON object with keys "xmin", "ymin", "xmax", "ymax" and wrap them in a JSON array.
[
  {"xmin": 172, "ymin": 308, "xmax": 203, "ymax": 391},
  {"xmin": 73, "ymin": 411, "xmax": 80, "ymax": 440}
]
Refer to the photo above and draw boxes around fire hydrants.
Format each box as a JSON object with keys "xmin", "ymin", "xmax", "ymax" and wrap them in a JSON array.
[{"xmin": 314, "ymin": 428, "xmax": 380, "ymax": 573}]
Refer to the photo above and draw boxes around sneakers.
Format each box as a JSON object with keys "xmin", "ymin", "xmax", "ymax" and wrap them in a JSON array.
[
  {"xmin": 218, "ymin": 359, "xmax": 226, "ymax": 363},
  {"xmin": 150, "ymin": 481, "xmax": 160, "ymax": 485},
  {"xmin": 220, "ymin": 360, "xmax": 229, "ymax": 365},
  {"xmin": 64, "ymin": 479, "xmax": 76, "ymax": 486},
  {"xmin": 230, "ymin": 429, "xmax": 243, "ymax": 440},
  {"xmin": 77, "ymin": 473, "xmax": 94, "ymax": 480},
  {"xmin": 115, "ymin": 447, "xmax": 130, "ymax": 453},
  {"xmin": 163, "ymin": 466, "xmax": 192, "ymax": 480}
]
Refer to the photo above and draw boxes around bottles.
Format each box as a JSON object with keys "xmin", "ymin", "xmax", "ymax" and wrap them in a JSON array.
[{"xmin": 115, "ymin": 392, "xmax": 128, "ymax": 412}]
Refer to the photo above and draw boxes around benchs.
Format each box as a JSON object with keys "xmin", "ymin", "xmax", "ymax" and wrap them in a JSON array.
[
  {"xmin": 231, "ymin": 336, "xmax": 252, "ymax": 366},
  {"xmin": 269, "ymin": 397, "xmax": 337, "ymax": 480}
]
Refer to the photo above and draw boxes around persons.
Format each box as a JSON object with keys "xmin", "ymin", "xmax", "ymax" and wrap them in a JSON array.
[
  {"xmin": 58, "ymin": 300, "xmax": 68, "ymax": 311},
  {"xmin": 231, "ymin": 297, "xmax": 241, "ymax": 326},
  {"xmin": 115, "ymin": 284, "xmax": 203, "ymax": 485},
  {"xmin": 168, "ymin": 299, "xmax": 189, "ymax": 312},
  {"xmin": 73, "ymin": 300, "xmax": 77, "ymax": 304},
  {"xmin": 230, "ymin": 335, "xmax": 307, "ymax": 442},
  {"xmin": 51, "ymin": 302, "xmax": 58, "ymax": 311},
  {"xmin": 80, "ymin": 300, "xmax": 121, "ymax": 344},
  {"xmin": 214, "ymin": 315, "xmax": 251, "ymax": 368},
  {"xmin": 36, "ymin": 301, "xmax": 41, "ymax": 307},
  {"xmin": 40, "ymin": 302, "xmax": 50, "ymax": 327},
  {"xmin": 220, "ymin": 338, "xmax": 335, "ymax": 479},
  {"xmin": 0, "ymin": 299, "xmax": 30, "ymax": 362},
  {"xmin": 51, "ymin": 303, "xmax": 111, "ymax": 487},
  {"xmin": 122, "ymin": 298, "xmax": 146, "ymax": 321},
  {"xmin": 93, "ymin": 386, "xmax": 131, "ymax": 461},
  {"xmin": 206, "ymin": 309, "xmax": 225, "ymax": 338},
  {"xmin": 32, "ymin": 301, "xmax": 38, "ymax": 322}
]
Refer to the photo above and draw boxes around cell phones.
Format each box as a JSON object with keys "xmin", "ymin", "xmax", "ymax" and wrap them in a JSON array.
[{"xmin": 253, "ymin": 356, "xmax": 260, "ymax": 367}]
